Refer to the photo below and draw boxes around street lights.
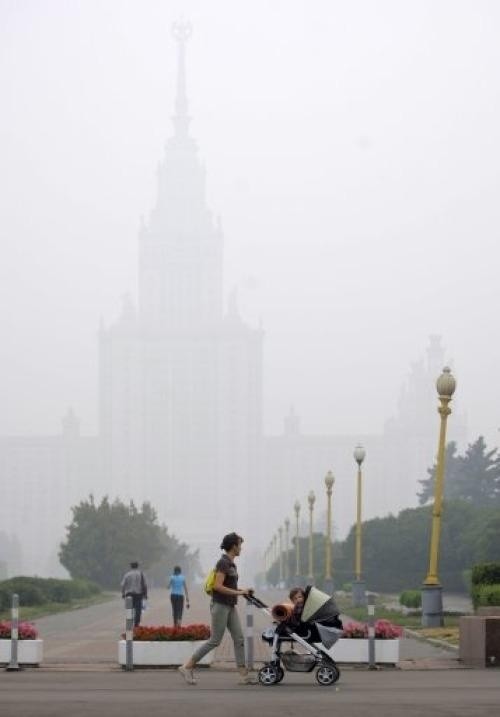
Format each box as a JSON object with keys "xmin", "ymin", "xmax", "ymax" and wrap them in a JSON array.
[
  {"xmin": 424, "ymin": 370, "xmax": 457, "ymax": 583},
  {"xmin": 263, "ymin": 490, "xmax": 316, "ymax": 580},
  {"xmin": 324, "ymin": 471, "xmax": 335, "ymax": 579},
  {"xmin": 353, "ymin": 443, "xmax": 366, "ymax": 582}
]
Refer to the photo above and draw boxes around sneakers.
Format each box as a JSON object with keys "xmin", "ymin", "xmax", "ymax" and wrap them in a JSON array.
[
  {"xmin": 179, "ymin": 663, "xmax": 194, "ymax": 683},
  {"xmin": 238, "ymin": 675, "xmax": 257, "ymax": 684}
]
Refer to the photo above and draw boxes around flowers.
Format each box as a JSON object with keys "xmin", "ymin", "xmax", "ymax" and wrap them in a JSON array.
[
  {"xmin": 341, "ymin": 620, "xmax": 402, "ymax": 640},
  {"xmin": 1, "ymin": 619, "xmax": 39, "ymax": 639},
  {"xmin": 119, "ymin": 623, "xmax": 209, "ymax": 641}
]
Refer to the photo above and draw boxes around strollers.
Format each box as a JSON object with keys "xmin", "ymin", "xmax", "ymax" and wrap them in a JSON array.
[{"xmin": 241, "ymin": 589, "xmax": 343, "ymax": 685}]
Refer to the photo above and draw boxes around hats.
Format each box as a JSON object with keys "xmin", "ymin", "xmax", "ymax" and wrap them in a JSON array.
[{"xmin": 130, "ymin": 561, "xmax": 137, "ymax": 567}]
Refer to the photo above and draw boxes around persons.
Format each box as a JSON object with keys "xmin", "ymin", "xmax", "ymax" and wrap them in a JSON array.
[
  {"xmin": 119, "ymin": 561, "xmax": 147, "ymax": 627},
  {"xmin": 167, "ymin": 566, "xmax": 188, "ymax": 626},
  {"xmin": 177, "ymin": 531, "xmax": 260, "ymax": 685},
  {"xmin": 289, "ymin": 586, "xmax": 304, "ymax": 614}
]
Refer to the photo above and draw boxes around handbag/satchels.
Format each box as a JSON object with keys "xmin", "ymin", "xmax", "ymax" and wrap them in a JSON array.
[{"xmin": 204, "ymin": 569, "xmax": 216, "ymax": 594}]
